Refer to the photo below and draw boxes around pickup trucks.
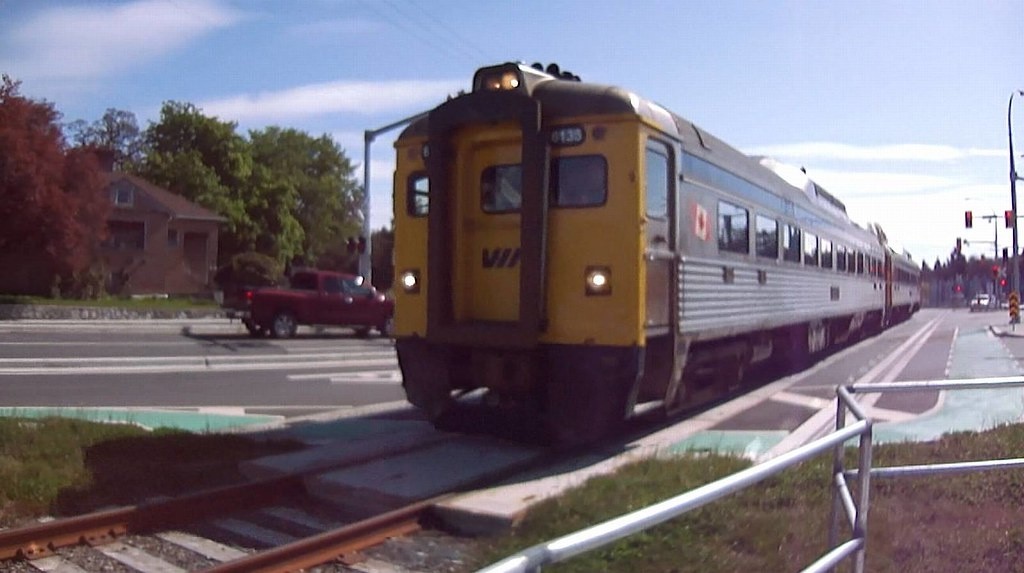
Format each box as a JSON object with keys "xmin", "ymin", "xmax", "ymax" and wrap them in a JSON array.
[{"xmin": 227, "ymin": 270, "xmax": 394, "ymax": 335}]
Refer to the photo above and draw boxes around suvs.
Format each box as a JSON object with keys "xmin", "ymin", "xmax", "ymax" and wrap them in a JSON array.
[{"xmin": 970, "ymin": 294, "xmax": 996, "ymax": 311}]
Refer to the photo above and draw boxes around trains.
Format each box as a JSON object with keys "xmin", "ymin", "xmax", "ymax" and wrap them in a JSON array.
[{"xmin": 392, "ymin": 60, "xmax": 922, "ymax": 441}]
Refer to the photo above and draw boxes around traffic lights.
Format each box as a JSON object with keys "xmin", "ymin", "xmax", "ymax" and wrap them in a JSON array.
[
  {"xmin": 1005, "ymin": 210, "xmax": 1015, "ymax": 228},
  {"xmin": 965, "ymin": 211, "xmax": 972, "ymax": 228}
]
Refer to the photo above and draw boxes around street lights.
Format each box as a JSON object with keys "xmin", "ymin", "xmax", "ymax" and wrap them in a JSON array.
[
  {"xmin": 982, "ymin": 215, "xmax": 998, "ymax": 294},
  {"xmin": 1008, "ymin": 89, "xmax": 1023, "ymax": 322}
]
